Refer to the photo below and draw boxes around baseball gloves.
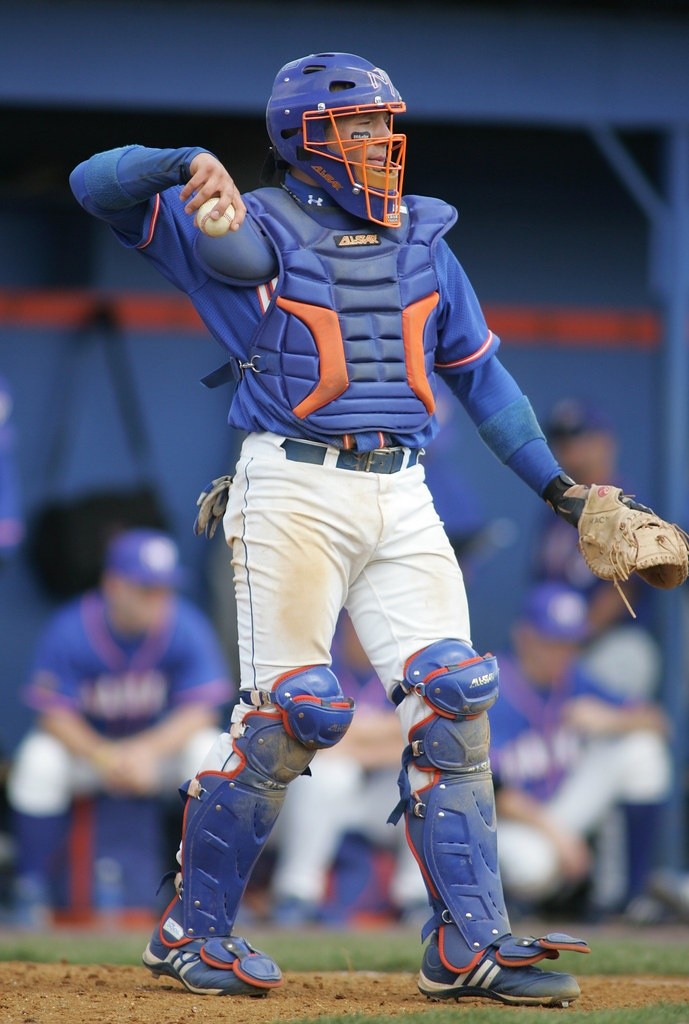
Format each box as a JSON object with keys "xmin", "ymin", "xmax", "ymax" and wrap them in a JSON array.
[{"xmin": 577, "ymin": 484, "xmax": 689, "ymax": 618}]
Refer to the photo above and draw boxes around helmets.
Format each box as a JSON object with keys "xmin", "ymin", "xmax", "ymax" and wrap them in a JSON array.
[{"xmin": 263, "ymin": 52, "xmax": 406, "ymax": 219}]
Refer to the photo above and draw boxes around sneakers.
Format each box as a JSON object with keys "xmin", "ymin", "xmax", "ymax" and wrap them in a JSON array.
[
  {"xmin": 142, "ymin": 920, "xmax": 270, "ymax": 998},
  {"xmin": 416, "ymin": 933, "xmax": 583, "ymax": 1007}
]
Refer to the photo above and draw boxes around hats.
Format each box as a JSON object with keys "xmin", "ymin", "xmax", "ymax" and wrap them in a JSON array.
[
  {"xmin": 103, "ymin": 525, "xmax": 202, "ymax": 592},
  {"xmin": 512, "ymin": 577, "xmax": 596, "ymax": 643}
]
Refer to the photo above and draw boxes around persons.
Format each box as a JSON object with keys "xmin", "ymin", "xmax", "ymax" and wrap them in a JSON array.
[
  {"xmin": 65, "ymin": 51, "xmax": 689, "ymax": 1008},
  {"xmin": 263, "ymin": 393, "xmax": 689, "ymax": 932},
  {"xmin": 2, "ymin": 526, "xmax": 242, "ymax": 946}
]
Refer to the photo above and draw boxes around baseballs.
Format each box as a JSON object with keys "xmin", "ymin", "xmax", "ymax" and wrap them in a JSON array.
[{"xmin": 197, "ymin": 198, "xmax": 235, "ymax": 238}]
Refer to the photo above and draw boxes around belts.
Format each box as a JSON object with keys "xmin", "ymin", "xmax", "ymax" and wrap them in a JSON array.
[{"xmin": 280, "ymin": 436, "xmax": 421, "ymax": 477}]
